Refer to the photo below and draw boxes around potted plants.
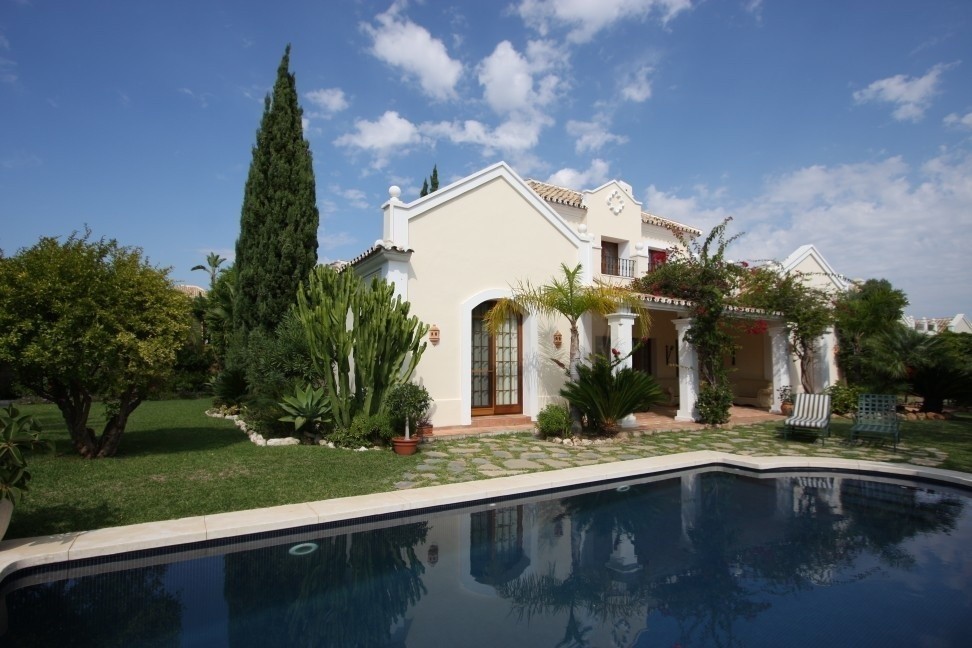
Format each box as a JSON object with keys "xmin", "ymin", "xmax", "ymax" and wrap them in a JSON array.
[
  {"xmin": 776, "ymin": 385, "xmax": 795, "ymax": 415},
  {"xmin": 0, "ymin": 401, "xmax": 47, "ymax": 548},
  {"xmin": 416, "ymin": 410, "xmax": 434, "ymax": 437},
  {"xmin": 383, "ymin": 382, "xmax": 433, "ymax": 457}
]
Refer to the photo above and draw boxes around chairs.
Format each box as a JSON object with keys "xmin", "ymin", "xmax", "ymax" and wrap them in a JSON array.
[
  {"xmin": 849, "ymin": 393, "xmax": 901, "ymax": 452},
  {"xmin": 784, "ymin": 393, "xmax": 832, "ymax": 447}
]
